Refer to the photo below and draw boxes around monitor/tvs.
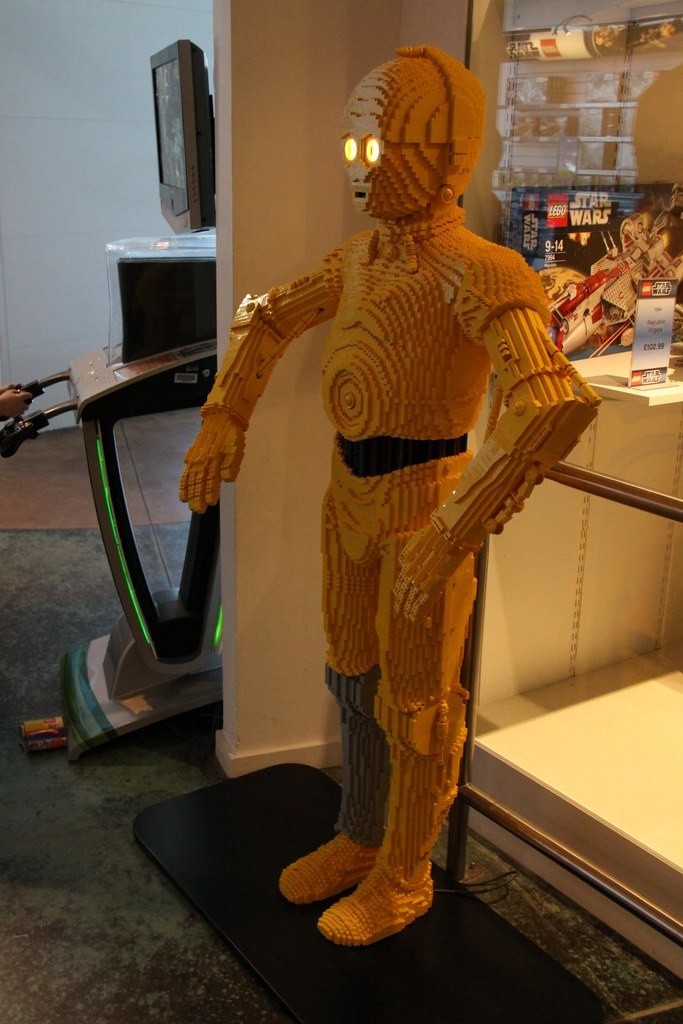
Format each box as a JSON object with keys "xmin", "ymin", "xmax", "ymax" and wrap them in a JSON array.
[{"xmin": 149, "ymin": 39, "xmax": 216, "ymax": 237}]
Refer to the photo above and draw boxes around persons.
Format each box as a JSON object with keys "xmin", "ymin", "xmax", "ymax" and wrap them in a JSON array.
[{"xmin": 180, "ymin": 44, "xmax": 602, "ymax": 945}]
